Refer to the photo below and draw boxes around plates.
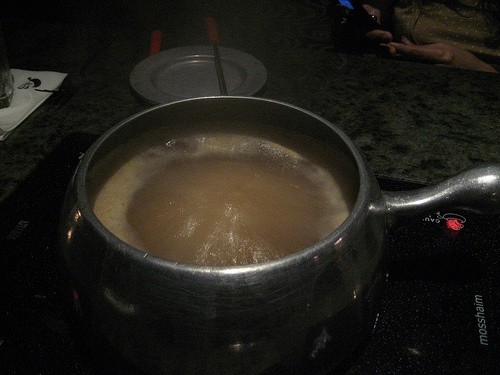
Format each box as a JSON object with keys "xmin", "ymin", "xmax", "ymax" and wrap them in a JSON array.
[
  {"xmin": 1, "ymin": 67, "xmax": 70, "ymax": 141},
  {"xmin": 129, "ymin": 45, "xmax": 269, "ymax": 103}
]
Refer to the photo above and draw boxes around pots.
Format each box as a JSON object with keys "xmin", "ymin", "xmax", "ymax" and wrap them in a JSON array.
[{"xmin": 61, "ymin": 89, "xmax": 500, "ymax": 341}]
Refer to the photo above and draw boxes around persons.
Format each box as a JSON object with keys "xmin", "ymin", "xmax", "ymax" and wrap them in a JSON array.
[{"xmin": 333, "ymin": 0, "xmax": 500, "ymax": 74}]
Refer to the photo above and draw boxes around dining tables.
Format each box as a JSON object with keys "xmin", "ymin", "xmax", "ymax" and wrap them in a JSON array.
[
  {"xmin": 0, "ymin": 65, "xmax": 72, "ymax": 146},
  {"xmin": 1, "ymin": 15, "xmax": 500, "ymax": 375}
]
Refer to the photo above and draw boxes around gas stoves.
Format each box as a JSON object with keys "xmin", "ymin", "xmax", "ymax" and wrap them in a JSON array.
[{"xmin": 1, "ymin": 115, "xmax": 500, "ymax": 375}]
